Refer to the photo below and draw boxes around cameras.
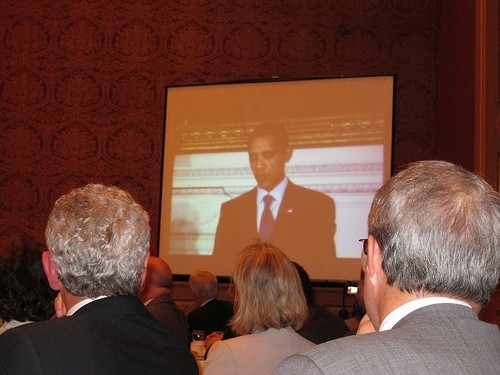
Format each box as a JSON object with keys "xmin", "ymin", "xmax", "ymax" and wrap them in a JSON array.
[{"xmin": 345, "ymin": 281, "xmax": 358, "ymax": 296}]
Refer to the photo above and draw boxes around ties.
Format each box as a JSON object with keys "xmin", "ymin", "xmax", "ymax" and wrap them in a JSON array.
[{"xmin": 257, "ymin": 195, "xmax": 277, "ymax": 241}]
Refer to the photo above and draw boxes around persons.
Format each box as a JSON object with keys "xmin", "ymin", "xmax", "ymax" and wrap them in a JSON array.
[
  {"xmin": 187, "ymin": 270, "xmax": 241, "ymax": 362},
  {"xmin": 1, "ymin": 183, "xmax": 199, "ymax": 374},
  {"xmin": 271, "ymin": 159, "xmax": 500, "ymax": 375},
  {"xmin": 290, "ymin": 236, "xmax": 378, "ymax": 345},
  {"xmin": 211, "ymin": 123, "xmax": 338, "ymax": 259},
  {"xmin": 1, "ymin": 236, "xmax": 60, "ymax": 336},
  {"xmin": 202, "ymin": 238, "xmax": 318, "ymax": 375},
  {"xmin": 137, "ymin": 255, "xmax": 190, "ymax": 351}
]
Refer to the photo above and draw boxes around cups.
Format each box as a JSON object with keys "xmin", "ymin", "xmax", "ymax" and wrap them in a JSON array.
[
  {"xmin": 189, "ymin": 330, "xmax": 206, "ymax": 345},
  {"xmin": 195, "ymin": 356, "xmax": 204, "ymax": 361}
]
